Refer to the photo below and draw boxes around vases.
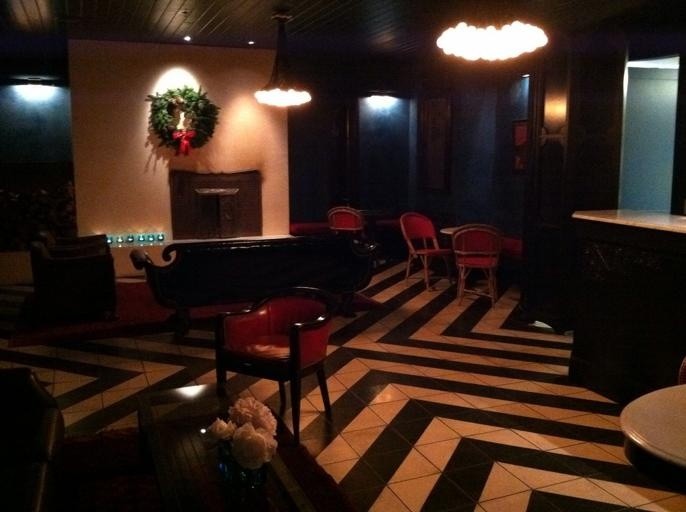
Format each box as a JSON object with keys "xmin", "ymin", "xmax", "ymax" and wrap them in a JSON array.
[{"xmin": 215, "ymin": 461, "xmax": 266, "ymax": 512}]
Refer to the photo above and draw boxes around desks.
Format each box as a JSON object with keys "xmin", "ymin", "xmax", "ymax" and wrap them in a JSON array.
[
  {"xmin": 439, "ymin": 224, "xmax": 523, "ymax": 287},
  {"xmin": 290, "ymin": 219, "xmax": 402, "ymax": 259}
]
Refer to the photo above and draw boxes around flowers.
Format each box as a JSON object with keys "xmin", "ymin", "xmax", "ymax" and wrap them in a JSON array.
[{"xmin": 211, "ymin": 398, "xmax": 278, "ymax": 469}]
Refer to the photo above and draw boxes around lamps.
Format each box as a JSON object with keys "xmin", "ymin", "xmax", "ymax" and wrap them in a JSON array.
[
  {"xmin": 254, "ymin": 15, "xmax": 313, "ymax": 108},
  {"xmin": 435, "ymin": 0, "xmax": 551, "ymax": 63}
]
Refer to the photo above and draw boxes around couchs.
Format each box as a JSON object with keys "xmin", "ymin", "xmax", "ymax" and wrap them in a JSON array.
[
  {"xmin": 130, "ymin": 229, "xmax": 322, "ymax": 322},
  {"xmin": 0, "ymin": 367, "xmax": 64, "ymax": 512}
]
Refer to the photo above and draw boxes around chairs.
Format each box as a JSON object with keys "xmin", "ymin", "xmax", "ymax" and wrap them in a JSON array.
[
  {"xmin": 399, "ymin": 212, "xmax": 452, "ymax": 291},
  {"xmin": 30, "ymin": 241, "xmax": 120, "ymax": 326},
  {"xmin": 327, "ymin": 206, "xmax": 389, "ymax": 269},
  {"xmin": 451, "ymin": 224, "xmax": 503, "ymax": 309},
  {"xmin": 38, "ymin": 228, "xmax": 107, "ymax": 248},
  {"xmin": 212, "ymin": 286, "xmax": 339, "ymax": 442}
]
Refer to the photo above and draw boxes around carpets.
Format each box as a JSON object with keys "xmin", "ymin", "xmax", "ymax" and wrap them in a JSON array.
[{"xmin": 49, "ymin": 403, "xmax": 359, "ymax": 512}]
